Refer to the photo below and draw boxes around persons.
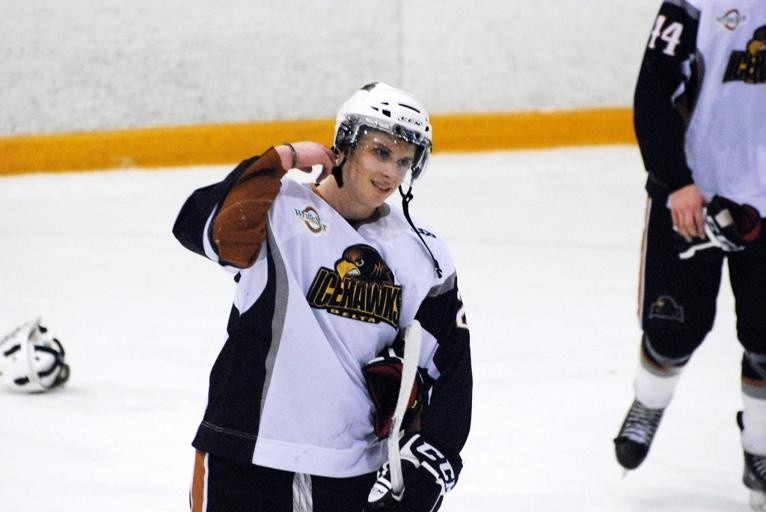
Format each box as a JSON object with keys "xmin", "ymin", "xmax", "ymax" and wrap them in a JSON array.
[
  {"xmin": 172, "ymin": 82, "xmax": 473, "ymax": 511},
  {"xmin": 612, "ymin": 0, "xmax": 766, "ymax": 493}
]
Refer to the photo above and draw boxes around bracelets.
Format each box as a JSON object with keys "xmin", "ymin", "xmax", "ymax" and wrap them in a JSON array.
[{"xmin": 284, "ymin": 143, "xmax": 297, "ymax": 170}]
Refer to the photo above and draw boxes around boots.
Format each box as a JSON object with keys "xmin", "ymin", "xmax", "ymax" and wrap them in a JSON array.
[
  {"xmin": 735, "ymin": 411, "xmax": 766, "ymax": 493},
  {"xmin": 613, "ymin": 393, "xmax": 667, "ymax": 469}
]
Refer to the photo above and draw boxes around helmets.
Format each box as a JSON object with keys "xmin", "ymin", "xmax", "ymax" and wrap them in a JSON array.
[
  {"xmin": 0, "ymin": 319, "xmax": 74, "ymax": 392},
  {"xmin": 334, "ymin": 79, "xmax": 433, "ymax": 182}
]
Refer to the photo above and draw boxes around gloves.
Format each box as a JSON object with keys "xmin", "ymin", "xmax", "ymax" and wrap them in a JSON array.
[
  {"xmin": 653, "ymin": 194, "xmax": 764, "ymax": 273},
  {"xmin": 363, "ymin": 433, "xmax": 466, "ymax": 511}
]
